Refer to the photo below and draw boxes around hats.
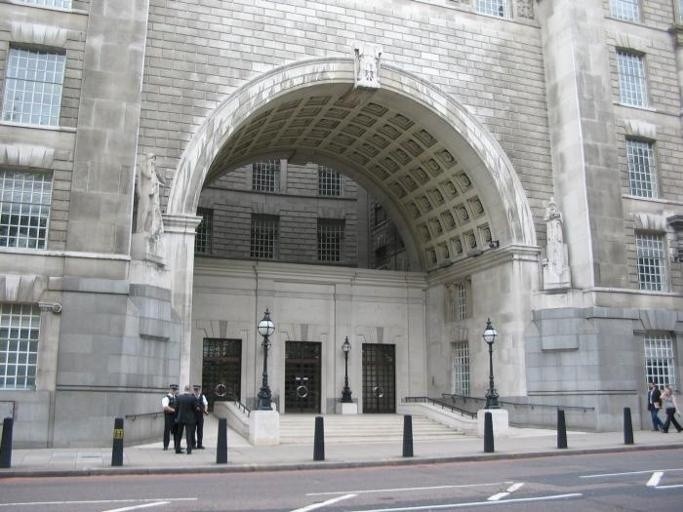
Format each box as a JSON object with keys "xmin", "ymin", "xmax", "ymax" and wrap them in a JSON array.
[
  {"xmin": 170, "ymin": 385, "xmax": 178, "ymax": 389},
  {"xmin": 193, "ymin": 385, "xmax": 200, "ymax": 389}
]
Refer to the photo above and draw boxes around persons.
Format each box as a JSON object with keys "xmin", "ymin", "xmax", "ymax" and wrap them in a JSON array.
[
  {"xmin": 191, "ymin": 385, "xmax": 209, "ymax": 449},
  {"xmin": 174, "ymin": 385, "xmax": 200, "ymax": 454},
  {"xmin": 161, "ymin": 385, "xmax": 178, "ymax": 451},
  {"xmin": 543, "ymin": 197, "xmax": 565, "ymax": 267},
  {"xmin": 659, "ymin": 386, "xmax": 683, "ymax": 433},
  {"xmin": 135, "ymin": 153, "xmax": 164, "ymax": 236},
  {"xmin": 647, "ymin": 383, "xmax": 664, "ymax": 431}
]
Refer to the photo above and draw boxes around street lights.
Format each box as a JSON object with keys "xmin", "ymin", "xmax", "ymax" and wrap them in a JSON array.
[
  {"xmin": 253, "ymin": 309, "xmax": 275, "ymax": 409},
  {"xmin": 482, "ymin": 318, "xmax": 498, "ymax": 409},
  {"xmin": 340, "ymin": 336, "xmax": 351, "ymax": 401}
]
